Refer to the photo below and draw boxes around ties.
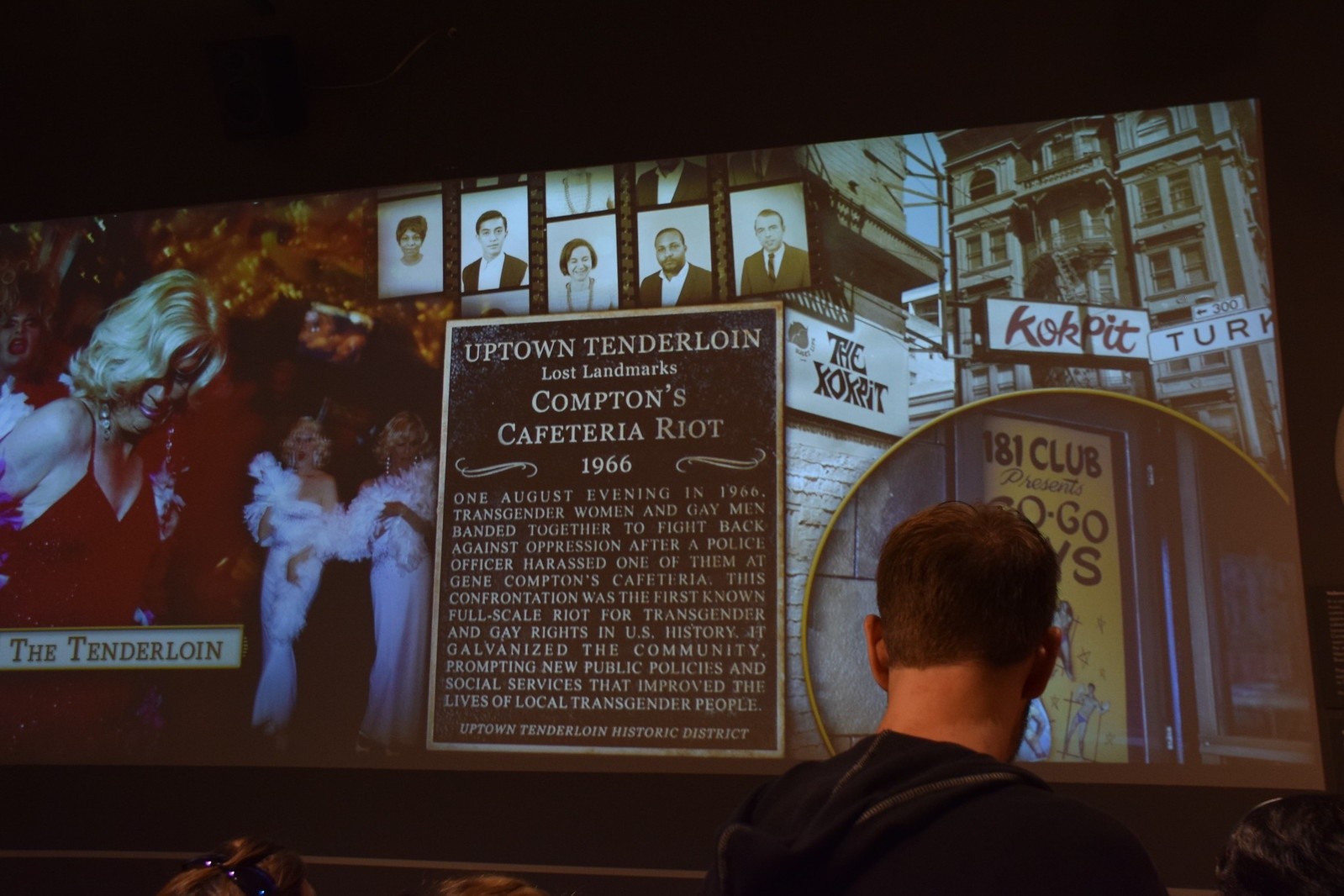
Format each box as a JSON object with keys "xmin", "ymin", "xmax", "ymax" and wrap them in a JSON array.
[{"xmin": 768, "ymin": 253, "xmax": 775, "ymax": 282}]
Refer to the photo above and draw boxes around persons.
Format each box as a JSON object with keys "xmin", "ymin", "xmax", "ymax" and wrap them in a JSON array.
[
  {"xmin": 726, "ymin": 148, "xmax": 793, "ymax": 188},
  {"xmin": 739, "ymin": 210, "xmax": 812, "ymax": 299},
  {"xmin": 156, "ymin": 837, "xmax": 316, "ymax": 896},
  {"xmin": 1216, "ymin": 795, "xmax": 1344, "ymax": 896},
  {"xmin": 640, "ymin": 227, "xmax": 712, "ymax": 310},
  {"xmin": 721, "ymin": 501, "xmax": 1174, "ymax": 896},
  {"xmin": 0, "ymin": 164, "xmax": 617, "ymax": 741},
  {"xmin": 636, "ymin": 155, "xmax": 710, "ymax": 206}
]
